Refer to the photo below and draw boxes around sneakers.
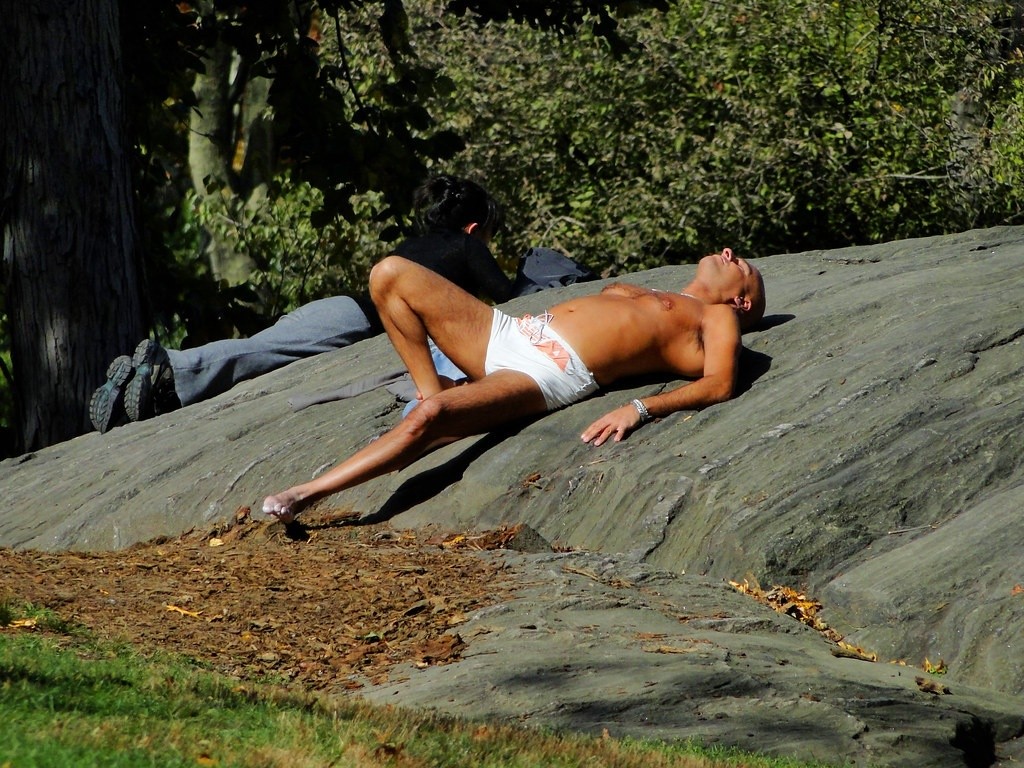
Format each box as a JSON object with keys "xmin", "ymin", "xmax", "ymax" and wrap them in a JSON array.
[
  {"xmin": 124, "ymin": 339, "xmax": 174, "ymax": 421},
  {"xmin": 89, "ymin": 355, "xmax": 136, "ymax": 434}
]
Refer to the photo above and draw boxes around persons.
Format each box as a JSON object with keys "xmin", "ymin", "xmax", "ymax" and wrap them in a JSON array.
[
  {"xmin": 262, "ymin": 247, "xmax": 766, "ymax": 524},
  {"xmin": 90, "ymin": 174, "xmax": 516, "ymax": 433}
]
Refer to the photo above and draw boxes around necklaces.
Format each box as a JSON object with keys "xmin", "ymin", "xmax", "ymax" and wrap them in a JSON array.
[{"xmin": 652, "ymin": 288, "xmax": 694, "ymax": 298}]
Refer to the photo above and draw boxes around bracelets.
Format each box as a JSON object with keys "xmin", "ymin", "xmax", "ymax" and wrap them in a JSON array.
[{"xmin": 630, "ymin": 398, "xmax": 652, "ymax": 422}]
